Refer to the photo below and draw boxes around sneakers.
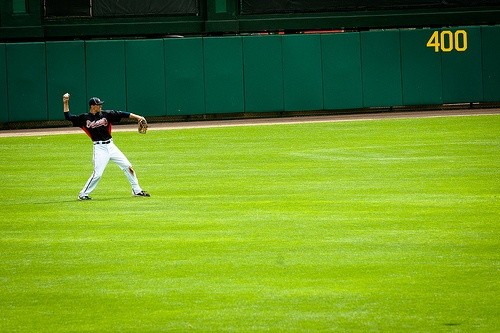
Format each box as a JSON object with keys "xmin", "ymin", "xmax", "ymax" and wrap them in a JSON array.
[
  {"xmin": 132, "ymin": 190, "xmax": 150, "ymax": 197},
  {"xmin": 78, "ymin": 196, "xmax": 92, "ymax": 200}
]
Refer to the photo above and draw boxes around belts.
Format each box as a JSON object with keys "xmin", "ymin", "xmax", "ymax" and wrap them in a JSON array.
[{"xmin": 94, "ymin": 141, "xmax": 110, "ymax": 145}]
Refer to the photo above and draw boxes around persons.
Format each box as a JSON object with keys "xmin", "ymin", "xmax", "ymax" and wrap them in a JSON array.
[{"xmin": 63, "ymin": 92, "xmax": 151, "ymax": 201}]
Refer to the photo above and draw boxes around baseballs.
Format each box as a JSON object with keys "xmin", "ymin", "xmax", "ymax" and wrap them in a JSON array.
[{"xmin": 63, "ymin": 94, "xmax": 69, "ymax": 99}]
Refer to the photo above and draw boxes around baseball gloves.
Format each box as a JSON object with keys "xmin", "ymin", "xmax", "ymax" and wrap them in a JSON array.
[{"xmin": 137, "ymin": 117, "xmax": 148, "ymax": 134}]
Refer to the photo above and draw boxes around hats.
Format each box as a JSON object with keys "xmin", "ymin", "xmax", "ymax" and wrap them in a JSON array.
[{"xmin": 89, "ymin": 98, "xmax": 104, "ymax": 105}]
